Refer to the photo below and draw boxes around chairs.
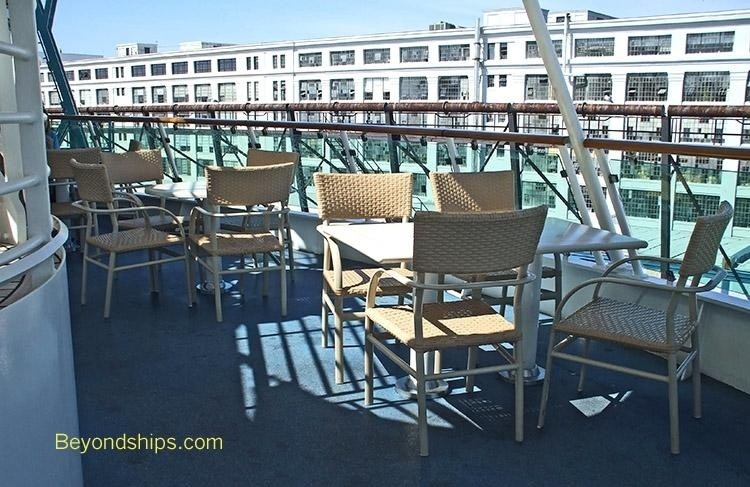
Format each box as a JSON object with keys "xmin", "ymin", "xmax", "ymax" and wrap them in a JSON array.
[
  {"xmin": 364, "ymin": 203, "xmax": 548, "ymax": 456},
  {"xmin": 46, "ymin": 146, "xmax": 299, "ymax": 323},
  {"xmin": 313, "ymin": 171, "xmax": 446, "ymax": 385},
  {"xmin": 430, "ymin": 168, "xmax": 563, "ymax": 366},
  {"xmin": 537, "ymin": 201, "xmax": 733, "ymax": 455}
]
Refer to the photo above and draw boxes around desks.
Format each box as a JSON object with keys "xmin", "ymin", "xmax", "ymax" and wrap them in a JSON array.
[{"xmin": 316, "ymin": 217, "xmax": 648, "ymax": 402}]
